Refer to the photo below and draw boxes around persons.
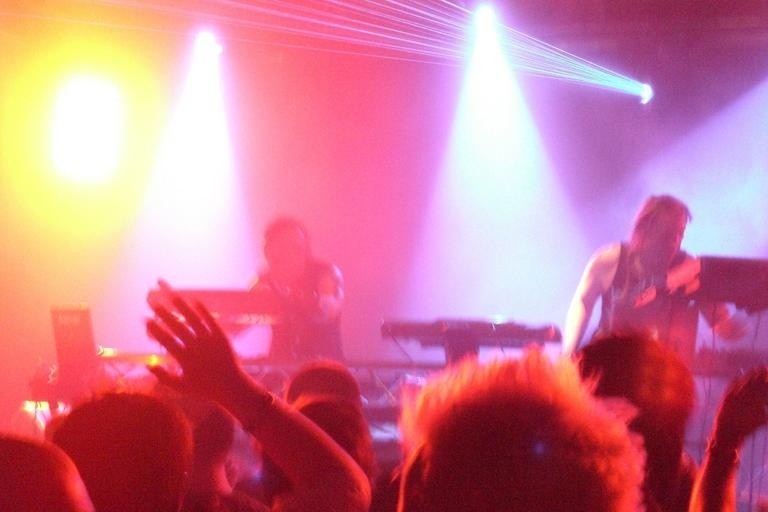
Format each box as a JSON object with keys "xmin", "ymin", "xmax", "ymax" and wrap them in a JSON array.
[
  {"xmin": 215, "ymin": 217, "xmax": 346, "ymax": 361},
  {"xmin": 560, "ymin": 193, "xmax": 754, "ymax": 362},
  {"xmin": 0, "ymin": 279, "xmax": 767, "ymax": 511}
]
